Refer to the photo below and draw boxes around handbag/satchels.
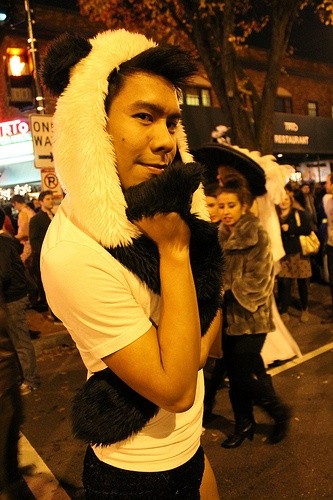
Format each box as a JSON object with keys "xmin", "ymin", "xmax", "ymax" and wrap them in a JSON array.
[{"xmin": 299, "ymin": 231, "xmax": 320, "ymax": 256}]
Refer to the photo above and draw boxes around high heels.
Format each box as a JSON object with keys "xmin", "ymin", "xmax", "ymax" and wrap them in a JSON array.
[
  {"xmin": 267, "ymin": 413, "xmax": 291, "ymax": 443},
  {"xmin": 221, "ymin": 422, "xmax": 256, "ymax": 449}
]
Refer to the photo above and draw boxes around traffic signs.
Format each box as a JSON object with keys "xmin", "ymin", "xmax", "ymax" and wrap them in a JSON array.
[{"xmin": 30, "ymin": 115, "xmax": 64, "ymax": 168}]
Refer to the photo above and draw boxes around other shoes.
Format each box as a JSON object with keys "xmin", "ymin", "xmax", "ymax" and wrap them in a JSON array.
[
  {"xmin": 28, "ymin": 330, "xmax": 42, "ymax": 339},
  {"xmin": 280, "ymin": 313, "xmax": 291, "ymax": 323},
  {"xmin": 301, "ymin": 307, "xmax": 310, "ymax": 323},
  {"xmin": 19, "ymin": 383, "xmax": 36, "ymax": 396}
]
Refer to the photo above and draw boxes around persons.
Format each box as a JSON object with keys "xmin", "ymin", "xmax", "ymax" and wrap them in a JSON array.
[
  {"xmin": 39, "ymin": 27, "xmax": 225, "ymax": 500},
  {"xmin": 0, "ymin": 144, "xmax": 333, "ymax": 500}
]
